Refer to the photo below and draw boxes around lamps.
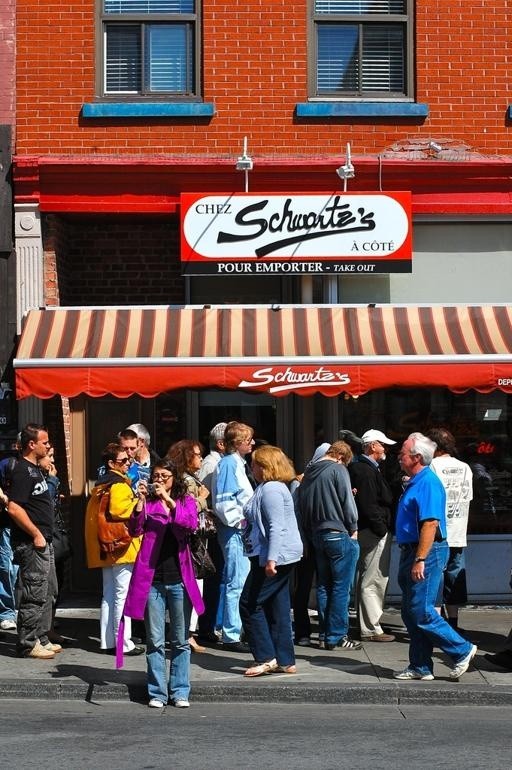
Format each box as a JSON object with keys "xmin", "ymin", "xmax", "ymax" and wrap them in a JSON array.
[
  {"xmin": 235, "ymin": 135, "xmax": 252, "ymax": 192},
  {"xmin": 335, "ymin": 142, "xmax": 355, "ymax": 191}
]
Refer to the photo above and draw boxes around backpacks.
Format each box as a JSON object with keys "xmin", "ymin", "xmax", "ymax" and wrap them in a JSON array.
[{"xmin": 95, "ymin": 483, "xmax": 132, "ymax": 562}]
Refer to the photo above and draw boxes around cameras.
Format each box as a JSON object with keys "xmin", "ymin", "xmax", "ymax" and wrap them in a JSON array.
[{"xmin": 147, "ymin": 484, "xmax": 156, "ymax": 493}]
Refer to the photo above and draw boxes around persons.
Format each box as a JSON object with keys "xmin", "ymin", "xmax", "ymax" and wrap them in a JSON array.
[
  {"xmin": 430, "ymin": 428, "xmax": 474, "ymax": 630},
  {"xmin": 291, "ymin": 429, "xmax": 410, "ymax": 650},
  {"xmin": 116, "ymin": 458, "xmax": 205, "ymax": 708},
  {"xmin": 392, "ymin": 432, "xmax": 477, "ymax": 680},
  {"xmin": 483, "ymin": 630, "xmax": 511, "ymax": 672},
  {"xmin": 0, "ymin": 422, "xmax": 73, "ymax": 661},
  {"xmin": 171, "ymin": 423, "xmax": 255, "ymax": 652},
  {"xmin": 239, "ymin": 444, "xmax": 303, "ymax": 677},
  {"xmin": 86, "ymin": 423, "xmax": 161, "ymax": 656}
]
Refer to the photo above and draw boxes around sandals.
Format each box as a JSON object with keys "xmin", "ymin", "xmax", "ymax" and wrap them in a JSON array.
[
  {"xmin": 269, "ymin": 665, "xmax": 297, "ymax": 674},
  {"xmin": 244, "ymin": 659, "xmax": 277, "ymax": 678}
]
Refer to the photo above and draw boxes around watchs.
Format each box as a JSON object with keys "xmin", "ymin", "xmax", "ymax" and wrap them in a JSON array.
[{"xmin": 414, "ymin": 557, "xmax": 425, "ymax": 562}]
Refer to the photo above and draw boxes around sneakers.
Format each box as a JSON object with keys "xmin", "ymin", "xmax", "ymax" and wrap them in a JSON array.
[
  {"xmin": 392, "ymin": 668, "xmax": 435, "ymax": 681},
  {"xmin": 175, "ymin": 701, "xmax": 191, "ymax": 708},
  {"xmin": 450, "ymin": 644, "xmax": 480, "ymax": 679},
  {"xmin": 53, "ymin": 621, "xmax": 59, "ymax": 627},
  {"xmin": 148, "ymin": 701, "xmax": 165, "ymax": 708},
  {"xmin": 1, "ymin": 620, "xmax": 18, "ymax": 630}
]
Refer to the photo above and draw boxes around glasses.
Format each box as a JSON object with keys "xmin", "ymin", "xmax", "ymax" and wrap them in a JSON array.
[
  {"xmin": 150, "ymin": 473, "xmax": 174, "ymax": 482},
  {"xmin": 113, "ymin": 456, "xmax": 131, "ymax": 464}
]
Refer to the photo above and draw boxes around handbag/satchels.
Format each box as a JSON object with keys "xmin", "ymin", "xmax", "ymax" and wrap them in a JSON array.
[
  {"xmin": 186, "ymin": 529, "xmax": 217, "ymax": 580},
  {"xmin": 197, "ymin": 508, "xmax": 218, "ymax": 539}
]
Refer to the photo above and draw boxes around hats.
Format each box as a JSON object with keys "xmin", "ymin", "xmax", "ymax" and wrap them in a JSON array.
[{"xmin": 362, "ymin": 429, "xmax": 398, "ymax": 447}]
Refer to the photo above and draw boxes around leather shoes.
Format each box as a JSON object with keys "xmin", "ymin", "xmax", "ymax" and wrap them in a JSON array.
[
  {"xmin": 43, "ymin": 642, "xmax": 62, "ymax": 653},
  {"xmin": 18, "ymin": 643, "xmax": 55, "ymax": 659},
  {"xmin": 189, "ymin": 629, "xmax": 396, "ymax": 653},
  {"xmin": 100, "ymin": 647, "xmax": 115, "ymax": 655},
  {"xmin": 484, "ymin": 649, "xmax": 512, "ymax": 670},
  {"xmin": 132, "ymin": 636, "xmax": 142, "ymax": 645},
  {"xmin": 123, "ymin": 647, "xmax": 145, "ymax": 657}
]
